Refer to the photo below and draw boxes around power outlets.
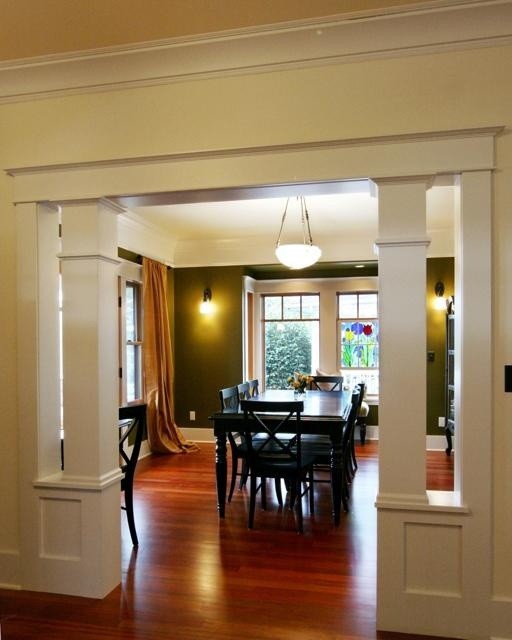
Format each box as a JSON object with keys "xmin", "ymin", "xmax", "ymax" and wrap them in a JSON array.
[
  {"xmin": 427, "ymin": 350, "xmax": 436, "ymax": 363},
  {"xmin": 190, "ymin": 411, "xmax": 195, "ymax": 420},
  {"xmin": 438, "ymin": 416, "xmax": 445, "ymax": 427}
]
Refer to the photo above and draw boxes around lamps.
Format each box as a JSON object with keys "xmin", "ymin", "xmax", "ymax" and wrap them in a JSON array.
[
  {"xmin": 274, "ymin": 197, "xmax": 321, "ymax": 271},
  {"xmin": 433, "ymin": 281, "xmax": 447, "ymax": 311}
]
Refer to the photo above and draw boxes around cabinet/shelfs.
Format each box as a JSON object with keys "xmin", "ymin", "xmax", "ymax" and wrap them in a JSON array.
[{"xmin": 444, "ymin": 295, "xmax": 455, "ymax": 453}]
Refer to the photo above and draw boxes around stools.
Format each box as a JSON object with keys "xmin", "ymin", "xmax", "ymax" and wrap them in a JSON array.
[{"xmin": 357, "ymin": 401, "xmax": 369, "ymax": 445}]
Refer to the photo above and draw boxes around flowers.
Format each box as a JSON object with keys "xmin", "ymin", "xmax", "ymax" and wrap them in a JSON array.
[{"xmin": 286, "ymin": 371, "xmax": 314, "ymax": 393}]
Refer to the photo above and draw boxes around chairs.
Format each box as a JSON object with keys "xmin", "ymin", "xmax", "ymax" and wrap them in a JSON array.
[
  {"xmin": 208, "ymin": 376, "xmax": 365, "ymax": 534},
  {"xmin": 119, "ymin": 402, "xmax": 148, "ymax": 546}
]
